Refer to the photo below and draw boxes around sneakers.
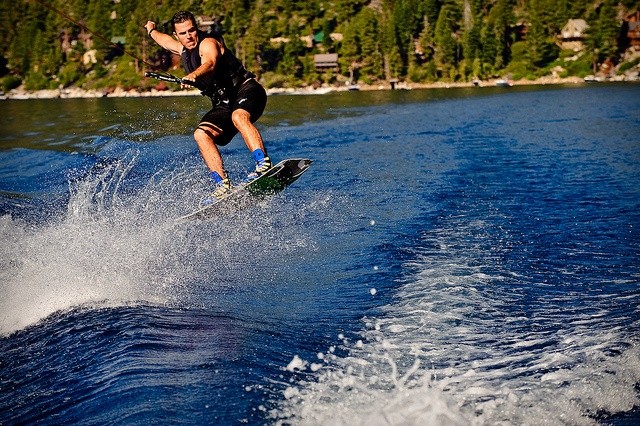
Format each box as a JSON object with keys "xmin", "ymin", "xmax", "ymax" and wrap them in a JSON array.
[
  {"xmin": 246, "ymin": 149, "xmax": 273, "ymax": 183},
  {"xmin": 199, "ymin": 171, "xmax": 232, "ymax": 206}
]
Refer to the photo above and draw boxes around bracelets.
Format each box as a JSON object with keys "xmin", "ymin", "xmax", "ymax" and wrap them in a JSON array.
[{"xmin": 149, "ymin": 27, "xmax": 158, "ymax": 39}]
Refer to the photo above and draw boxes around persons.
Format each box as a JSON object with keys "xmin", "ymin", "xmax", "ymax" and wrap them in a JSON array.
[{"xmin": 142, "ymin": 9, "xmax": 275, "ymax": 207}]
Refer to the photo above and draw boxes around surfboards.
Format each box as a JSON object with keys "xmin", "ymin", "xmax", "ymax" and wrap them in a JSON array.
[{"xmin": 173, "ymin": 158, "xmax": 313, "ymax": 221}]
